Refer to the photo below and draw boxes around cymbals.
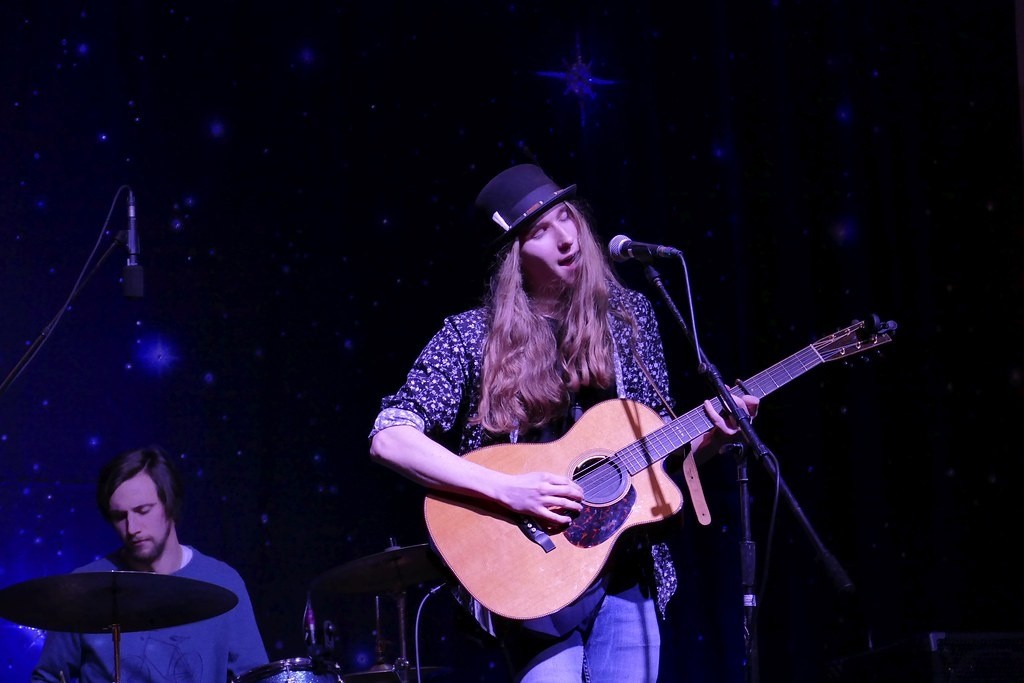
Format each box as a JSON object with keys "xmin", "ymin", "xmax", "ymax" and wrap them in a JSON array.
[
  {"xmin": 0, "ymin": 571, "xmax": 238, "ymax": 634},
  {"xmin": 310, "ymin": 543, "xmax": 433, "ymax": 596},
  {"xmin": 341, "ymin": 664, "xmax": 446, "ymax": 682}
]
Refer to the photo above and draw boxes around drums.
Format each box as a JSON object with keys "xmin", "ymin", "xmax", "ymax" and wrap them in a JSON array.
[{"xmin": 229, "ymin": 656, "xmax": 343, "ymax": 683}]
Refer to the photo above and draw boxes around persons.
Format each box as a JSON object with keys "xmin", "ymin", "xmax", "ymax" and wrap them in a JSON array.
[
  {"xmin": 368, "ymin": 165, "xmax": 760, "ymax": 683},
  {"xmin": 31, "ymin": 448, "xmax": 270, "ymax": 683}
]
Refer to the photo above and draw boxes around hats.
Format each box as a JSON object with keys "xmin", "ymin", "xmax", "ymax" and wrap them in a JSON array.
[{"xmin": 475, "ymin": 163, "xmax": 576, "ymax": 254}]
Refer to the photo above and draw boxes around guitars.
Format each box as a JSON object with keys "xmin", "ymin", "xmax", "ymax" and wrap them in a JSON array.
[{"xmin": 421, "ymin": 313, "xmax": 901, "ymax": 652}]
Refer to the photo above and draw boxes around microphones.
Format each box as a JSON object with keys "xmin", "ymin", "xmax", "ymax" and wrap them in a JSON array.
[
  {"xmin": 610, "ymin": 235, "xmax": 684, "ymax": 262},
  {"xmin": 307, "ymin": 599, "xmax": 317, "ymax": 645},
  {"xmin": 125, "ymin": 190, "xmax": 144, "ymax": 298}
]
